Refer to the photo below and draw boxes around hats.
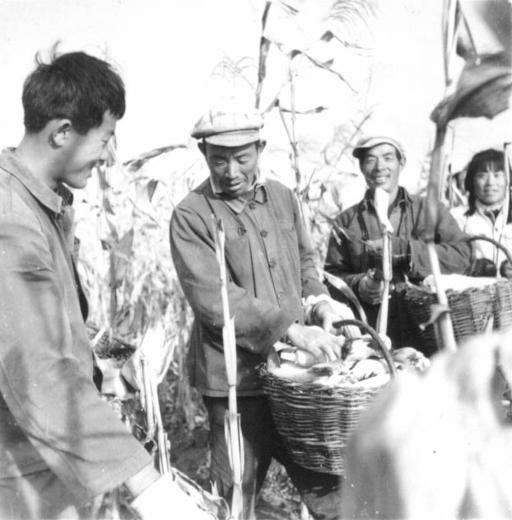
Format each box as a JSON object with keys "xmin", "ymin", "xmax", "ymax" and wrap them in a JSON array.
[
  {"xmin": 352, "ymin": 134, "xmax": 409, "ymax": 167},
  {"xmin": 189, "ymin": 104, "xmax": 264, "ymax": 145}
]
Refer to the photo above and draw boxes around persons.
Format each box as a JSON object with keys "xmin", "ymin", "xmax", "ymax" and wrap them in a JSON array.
[
  {"xmin": 447, "ymin": 149, "xmax": 512, "ymax": 276},
  {"xmin": 324, "ymin": 134, "xmax": 472, "ymax": 348},
  {"xmin": 0, "ymin": 40, "xmax": 218, "ymax": 520},
  {"xmin": 169, "ymin": 107, "xmax": 346, "ymax": 520}
]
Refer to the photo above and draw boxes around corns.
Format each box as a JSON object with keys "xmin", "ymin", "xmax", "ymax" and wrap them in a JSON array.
[{"xmin": 274, "ymin": 338, "xmax": 426, "ymax": 388}]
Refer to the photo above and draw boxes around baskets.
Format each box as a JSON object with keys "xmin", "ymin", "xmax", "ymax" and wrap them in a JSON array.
[
  {"xmin": 400, "ymin": 233, "xmax": 512, "ymax": 359},
  {"xmin": 256, "ymin": 321, "xmax": 429, "ymax": 474}
]
